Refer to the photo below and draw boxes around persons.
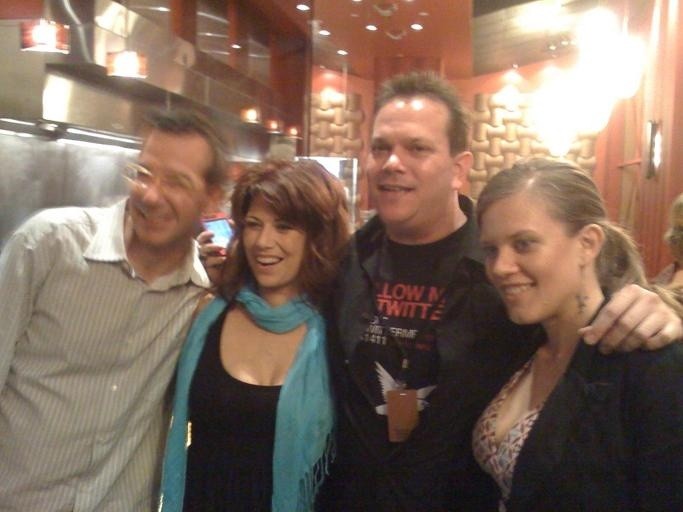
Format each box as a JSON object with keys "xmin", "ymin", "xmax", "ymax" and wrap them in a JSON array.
[
  {"xmin": 652, "ymin": 194, "xmax": 683, "ymax": 304},
  {"xmin": 159, "ymin": 153, "xmax": 349, "ymax": 512},
  {"xmin": 198, "ymin": 75, "xmax": 683, "ymax": 510},
  {"xmin": 472, "ymin": 159, "xmax": 683, "ymax": 511},
  {"xmin": 1, "ymin": 108, "xmax": 232, "ymax": 512}
]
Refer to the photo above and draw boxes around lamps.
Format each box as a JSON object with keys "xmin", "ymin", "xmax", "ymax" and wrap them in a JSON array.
[
  {"xmin": 241, "ymin": 106, "xmax": 302, "ymax": 140},
  {"xmin": 19, "ymin": 1, "xmax": 71, "ymax": 54},
  {"xmin": 106, "ymin": 8, "xmax": 147, "ymax": 79}
]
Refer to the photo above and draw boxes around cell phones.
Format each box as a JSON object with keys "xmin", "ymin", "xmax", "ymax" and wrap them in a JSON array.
[{"xmin": 200, "ymin": 217, "xmax": 235, "ymax": 248}]
